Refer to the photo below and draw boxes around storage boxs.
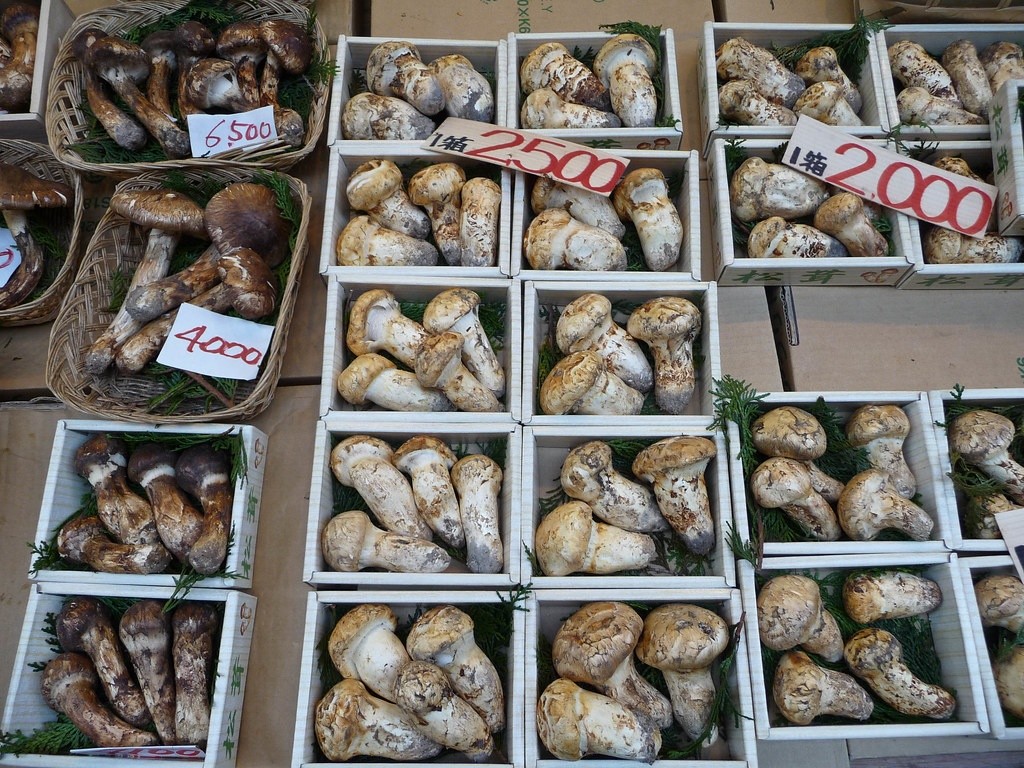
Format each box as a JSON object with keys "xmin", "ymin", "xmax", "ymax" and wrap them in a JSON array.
[
  {"xmin": 0, "ymin": 384, "xmax": 320, "ymax": 768},
  {"xmin": 0, "ymin": 0, "xmax": 77, "ymax": 139},
  {"xmin": 370, "ymin": 0, "xmax": 716, "ymax": 179},
  {"xmin": 0, "ymin": 43, "xmax": 330, "ymax": 400},
  {"xmin": 291, "ymin": 590, "xmax": 524, "ymax": 768},
  {"xmin": 0, "ymin": 582, "xmax": 258, "ymax": 768},
  {"xmin": 958, "ymin": 556, "xmax": 1024, "ymax": 742},
  {"xmin": 525, "ymin": 588, "xmax": 760, "ymax": 768},
  {"xmin": 737, "ymin": 551, "xmax": 991, "ymax": 742},
  {"xmin": 27, "ymin": 417, "xmax": 269, "ymax": 590},
  {"xmin": 770, "ymin": 286, "xmax": 1024, "ymax": 393},
  {"xmin": 302, "ymin": 22, "xmax": 1024, "ymax": 590}
]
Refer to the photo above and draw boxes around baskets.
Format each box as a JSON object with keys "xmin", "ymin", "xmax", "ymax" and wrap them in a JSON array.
[
  {"xmin": 0, "ymin": 139, "xmax": 86, "ymax": 328},
  {"xmin": 44, "ymin": 166, "xmax": 312, "ymax": 425},
  {"xmin": 44, "ymin": 0, "xmax": 330, "ymax": 181}
]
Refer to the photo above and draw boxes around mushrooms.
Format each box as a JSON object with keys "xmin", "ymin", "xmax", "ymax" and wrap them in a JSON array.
[
  {"xmin": 0, "ymin": 0, "xmax": 74, "ymax": 310},
  {"xmin": 52, "ymin": 435, "xmax": 234, "ymax": 573},
  {"xmin": 314, "ymin": 34, "xmax": 1024, "ymax": 764},
  {"xmin": 83, "ymin": 182, "xmax": 292, "ymax": 372},
  {"xmin": 40, "ymin": 596, "xmax": 218, "ymax": 750},
  {"xmin": 75, "ymin": 18, "xmax": 314, "ymax": 156}
]
